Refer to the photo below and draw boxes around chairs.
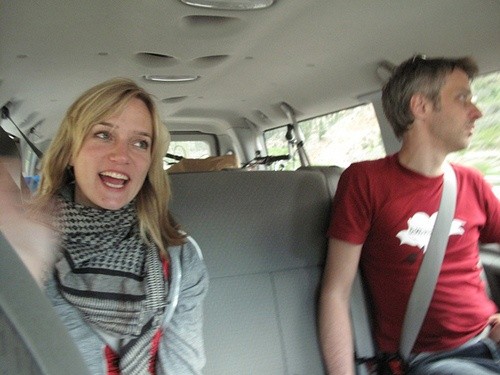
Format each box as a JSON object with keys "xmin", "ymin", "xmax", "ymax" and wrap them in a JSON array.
[{"xmin": 157, "ymin": 165, "xmax": 394, "ymax": 375}]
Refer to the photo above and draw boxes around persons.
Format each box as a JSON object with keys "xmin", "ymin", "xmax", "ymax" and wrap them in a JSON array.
[
  {"xmin": 313, "ymin": 51, "xmax": 500, "ymax": 374},
  {"xmin": 0, "ymin": 77, "xmax": 212, "ymax": 374}
]
